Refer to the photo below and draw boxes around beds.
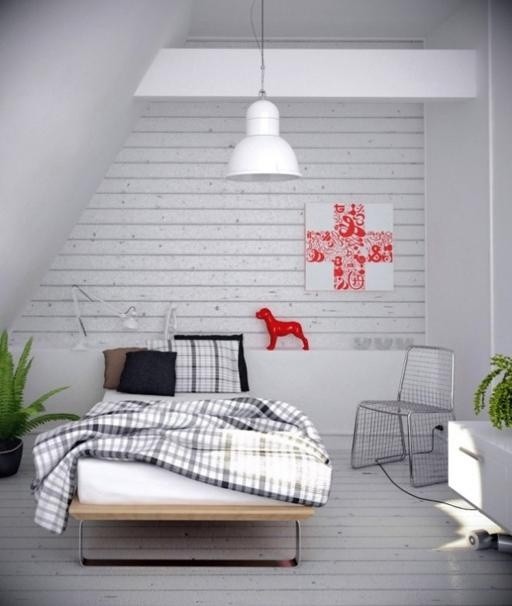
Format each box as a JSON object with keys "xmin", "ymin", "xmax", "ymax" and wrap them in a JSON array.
[{"xmin": 67, "ymin": 389, "xmax": 331, "ymax": 568}]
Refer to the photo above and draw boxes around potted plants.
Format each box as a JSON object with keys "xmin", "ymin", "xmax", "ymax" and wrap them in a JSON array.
[{"xmin": 0, "ymin": 328, "xmax": 82, "ymax": 478}]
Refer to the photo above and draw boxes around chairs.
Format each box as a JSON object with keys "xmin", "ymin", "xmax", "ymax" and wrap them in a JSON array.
[{"xmin": 349, "ymin": 344, "xmax": 455, "ymax": 487}]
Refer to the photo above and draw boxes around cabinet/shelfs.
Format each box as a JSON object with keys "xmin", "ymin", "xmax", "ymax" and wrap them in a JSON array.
[{"xmin": 443, "ymin": 420, "xmax": 511, "ymax": 537}]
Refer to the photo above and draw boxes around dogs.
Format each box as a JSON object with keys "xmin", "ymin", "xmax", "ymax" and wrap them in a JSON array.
[{"xmin": 256, "ymin": 308, "xmax": 309, "ymax": 350}]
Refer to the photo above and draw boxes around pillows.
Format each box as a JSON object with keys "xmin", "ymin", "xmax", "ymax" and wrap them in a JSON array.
[{"xmin": 102, "ymin": 333, "xmax": 249, "ymax": 396}]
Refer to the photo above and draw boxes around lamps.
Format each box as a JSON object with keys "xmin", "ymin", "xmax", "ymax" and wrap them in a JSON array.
[
  {"xmin": 71, "ymin": 283, "xmax": 139, "ymax": 352},
  {"xmin": 226, "ymin": 0, "xmax": 303, "ymax": 181}
]
